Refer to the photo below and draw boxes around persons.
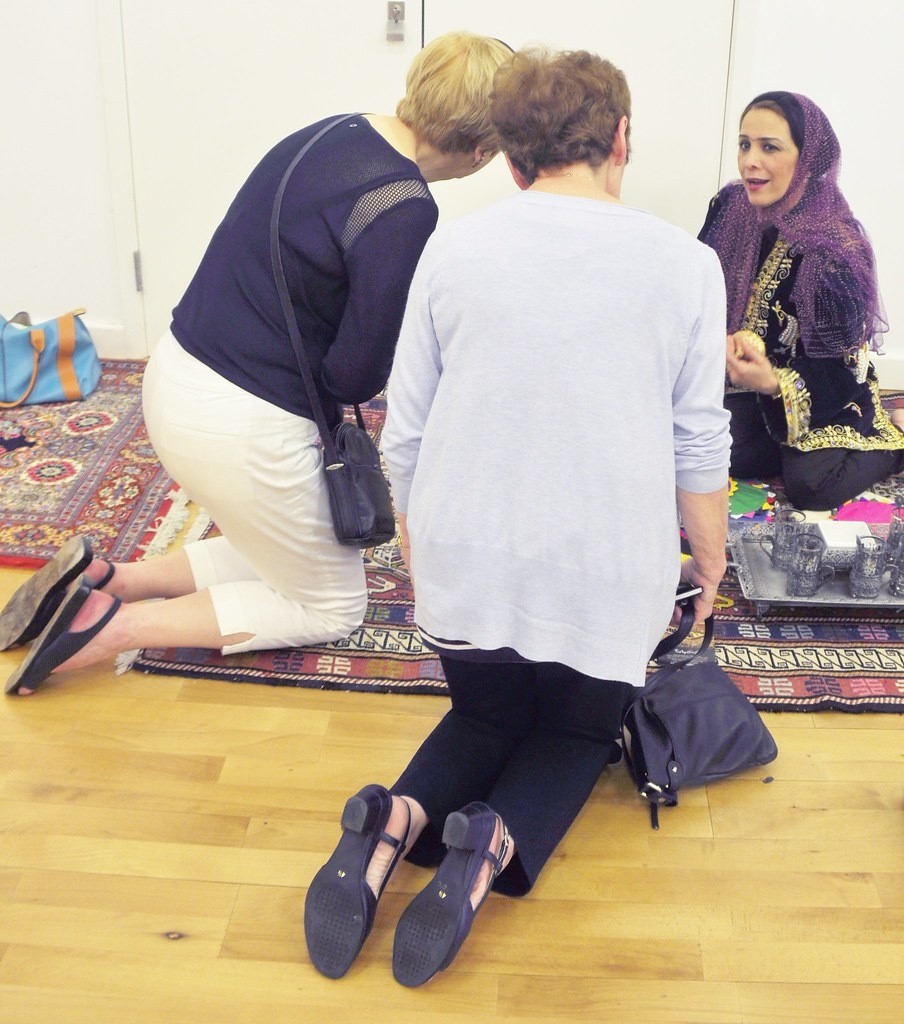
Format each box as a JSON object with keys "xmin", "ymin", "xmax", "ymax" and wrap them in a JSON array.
[
  {"xmin": 0, "ymin": 33, "xmax": 518, "ymax": 696},
  {"xmin": 690, "ymin": 85, "xmax": 904, "ymax": 515},
  {"xmin": 301, "ymin": 38, "xmax": 734, "ymax": 988}
]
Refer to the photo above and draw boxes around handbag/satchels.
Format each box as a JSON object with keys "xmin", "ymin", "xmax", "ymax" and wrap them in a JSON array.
[
  {"xmin": 323, "ymin": 421, "xmax": 395, "ymax": 548},
  {"xmin": 620, "ymin": 581, "xmax": 779, "ymax": 829},
  {"xmin": 0, "ymin": 308, "xmax": 102, "ymax": 408}
]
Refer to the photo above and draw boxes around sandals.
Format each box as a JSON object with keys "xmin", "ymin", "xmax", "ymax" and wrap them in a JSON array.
[
  {"xmin": 0, "ymin": 538, "xmax": 116, "ymax": 653},
  {"xmin": 4, "ymin": 573, "xmax": 121, "ymax": 692}
]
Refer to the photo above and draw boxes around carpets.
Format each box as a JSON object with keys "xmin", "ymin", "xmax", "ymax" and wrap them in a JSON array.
[
  {"xmin": 111, "ymin": 376, "xmax": 904, "ymax": 714},
  {"xmin": 0, "ymin": 358, "xmax": 190, "ymax": 572}
]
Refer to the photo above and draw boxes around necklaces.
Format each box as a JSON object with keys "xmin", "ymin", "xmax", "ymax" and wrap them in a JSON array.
[{"xmin": 532, "ymin": 171, "xmax": 593, "ymax": 184}]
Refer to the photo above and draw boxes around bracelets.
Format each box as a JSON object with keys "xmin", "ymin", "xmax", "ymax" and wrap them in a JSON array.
[{"xmin": 771, "ymin": 392, "xmax": 783, "ymax": 403}]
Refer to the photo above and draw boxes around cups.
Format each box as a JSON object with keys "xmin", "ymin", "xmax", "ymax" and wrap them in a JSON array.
[
  {"xmin": 760, "ymin": 510, "xmax": 807, "ymax": 570},
  {"xmin": 786, "ymin": 534, "xmax": 835, "ymax": 597},
  {"xmin": 885, "ymin": 505, "xmax": 904, "ymax": 564},
  {"xmin": 889, "ymin": 536, "xmax": 904, "ymax": 597},
  {"xmin": 850, "ymin": 536, "xmax": 898, "ymax": 598}
]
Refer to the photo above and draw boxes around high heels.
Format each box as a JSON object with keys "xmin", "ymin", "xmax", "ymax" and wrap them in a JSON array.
[
  {"xmin": 392, "ymin": 800, "xmax": 508, "ymax": 987},
  {"xmin": 304, "ymin": 784, "xmax": 412, "ymax": 980}
]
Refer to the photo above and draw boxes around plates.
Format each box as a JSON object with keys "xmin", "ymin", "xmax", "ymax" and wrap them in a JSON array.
[{"xmin": 817, "ymin": 521, "xmax": 877, "ymax": 548}]
